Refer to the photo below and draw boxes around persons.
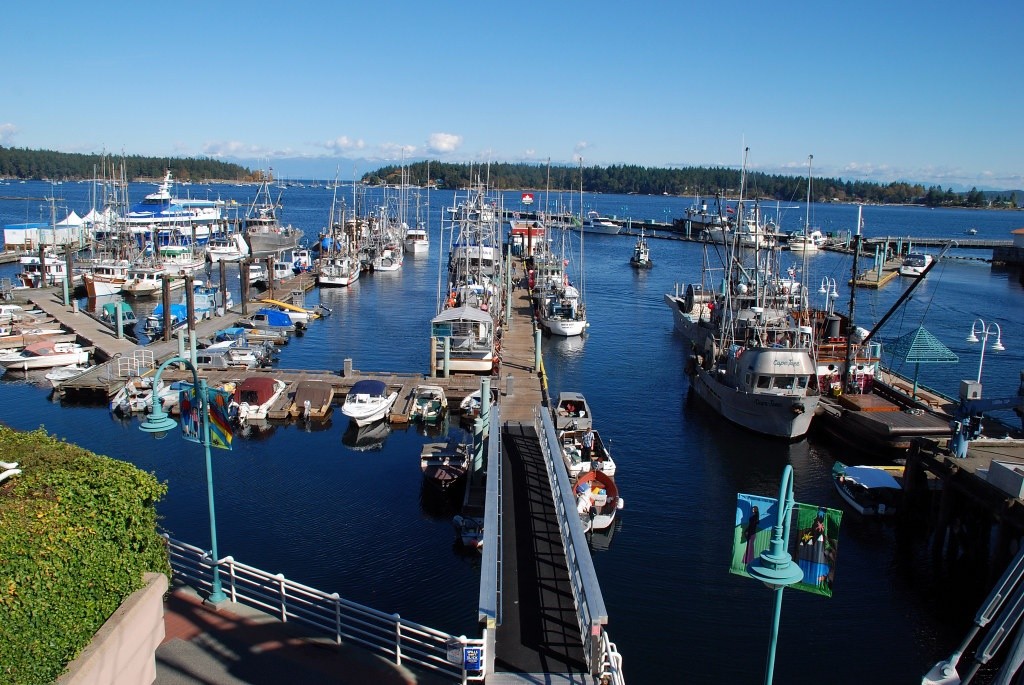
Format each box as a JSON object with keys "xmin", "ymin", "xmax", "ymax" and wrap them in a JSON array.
[
  {"xmin": 565, "ymin": 401, "xmax": 574, "ymax": 412},
  {"xmin": 581, "ymin": 426, "xmax": 595, "ymax": 464}
]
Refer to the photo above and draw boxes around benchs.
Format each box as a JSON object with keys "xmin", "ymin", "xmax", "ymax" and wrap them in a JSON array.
[
  {"xmin": 913, "ymin": 392, "xmax": 940, "ymax": 408},
  {"xmin": 891, "ymin": 382, "xmax": 913, "ymax": 394}
]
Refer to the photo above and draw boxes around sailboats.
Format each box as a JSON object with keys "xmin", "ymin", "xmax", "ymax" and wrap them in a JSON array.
[
  {"xmin": 534, "ymin": 155, "xmax": 584, "ymax": 337},
  {"xmin": 17, "ymin": 147, "xmax": 977, "ymax": 358}
]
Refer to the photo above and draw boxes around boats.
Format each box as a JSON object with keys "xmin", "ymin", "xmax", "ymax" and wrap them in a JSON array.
[
  {"xmin": 833, "ymin": 460, "xmax": 904, "ymax": 518},
  {"xmin": 144, "ymin": 379, "xmax": 195, "ymax": 414},
  {"xmin": 665, "ymin": 243, "xmax": 715, "ymax": 346},
  {"xmin": 340, "ymin": 420, "xmax": 392, "ymax": 450},
  {"xmin": 430, "ymin": 302, "xmax": 495, "ymax": 374},
  {"xmin": 459, "ymin": 386, "xmax": 495, "ymax": 427},
  {"xmin": 0, "ymin": 340, "xmax": 93, "ymax": 371},
  {"xmin": 110, "ymin": 376, "xmax": 164, "ymax": 413},
  {"xmin": 409, "ymin": 383, "xmax": 448, "ymax": 428},
  {"xmin": 570, "ymin": 467, "xmax": 623, "ymax": 534},
  {"xmin": 452, "ymin": 514, "xmax": 484, "ymax": 556},
  {"xmin": 228, "ymin": 375, "xmax": 287, "ymax": 421},
  {"xmin": 553, "ymin": 391, "xmax": 592, "ymax": 431},
  {"xmin": 554, "ymin": 429, "xmax": 617, "ymax": 477},
  {"xmin": 628, "ymin": 225, "xmax": 653, "ymax": 270},
  {"xmin": 689, "ymin": 144, "xmax": 821, "ymax": 443},
  {"xmin": 288, "ymin": 379, "xmax": 335, "ymax": 417},
  {"xmin": 0, "ymin": 326, "xmax": 66, "ymax": 336},
  {"xmin": 44, "ymin": 363, "xmax": 96, "ymax": 388},
  {"xmin": 342, "ymin": 379, "xmax": 398, "ymax": 427},
  {"xmin": 420, "ymin": 443, "xmax": 469, "ymax": 496},
  {"xmin": 0, "ymin": 304, "xmax": 44, "ymax": 319}
]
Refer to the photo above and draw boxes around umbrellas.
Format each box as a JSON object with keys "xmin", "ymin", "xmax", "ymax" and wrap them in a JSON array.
[{"xmin": 884, "ymin": 325, "xmax": 960, "ymax": 399}]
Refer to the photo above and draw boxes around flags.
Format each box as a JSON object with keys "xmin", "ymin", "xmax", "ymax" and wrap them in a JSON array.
[{"xmin": 727, "ymin": 205, "xmax": 734, "ymax": 213}]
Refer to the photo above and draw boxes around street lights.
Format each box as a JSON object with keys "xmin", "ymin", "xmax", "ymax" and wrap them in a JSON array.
[
  {"xmin": 747, "ymin": 464, "xmax": 805, "ymax": 685},
  {"xmin": 965, "ymin": 317, "xmax": 1006, "ymax": 384},
  {"xmin": 136, "ymin": 359, "xmax": 227, "ymax": 602}
]
